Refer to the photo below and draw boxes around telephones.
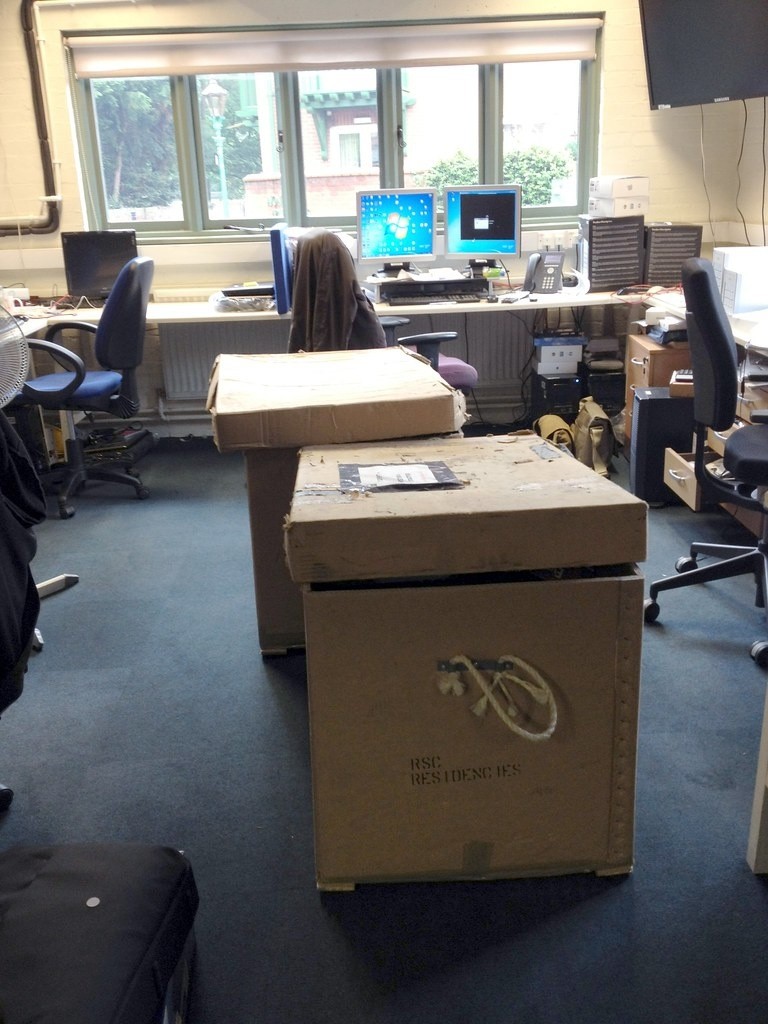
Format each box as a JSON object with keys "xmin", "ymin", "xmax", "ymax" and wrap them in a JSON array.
[{"xmin": 523, "ymin": 251, "xmax": 565, "ymax": 294}]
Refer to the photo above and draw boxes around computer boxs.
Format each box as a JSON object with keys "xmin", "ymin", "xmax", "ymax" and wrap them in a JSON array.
[
  {"xmin": 629, "ymin": 387, "xmax": 697, "ymax": 502},
  {"xmin": 531, "ymin": 368, "xmax": 626, "ymax": 426}
]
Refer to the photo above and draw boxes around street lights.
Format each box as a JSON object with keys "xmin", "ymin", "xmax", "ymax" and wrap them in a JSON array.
[{"xmin": 200, "ymin": 78, "xmax": 231, "ymax": 219}]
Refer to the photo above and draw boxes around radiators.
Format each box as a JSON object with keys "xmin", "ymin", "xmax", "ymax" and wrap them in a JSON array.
[{"xmin": 157, "ymin": 310, "xmax": 544, "ymax": 401}]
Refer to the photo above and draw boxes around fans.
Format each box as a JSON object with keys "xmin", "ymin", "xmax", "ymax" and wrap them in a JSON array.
[{"xmin": 0, "ymin": 306, "xmax": 80, "ymax": 652}]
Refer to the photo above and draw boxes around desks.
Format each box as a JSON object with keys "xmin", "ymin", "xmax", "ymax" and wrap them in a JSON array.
[{"xmin": 0, "ymin": 288, "xmax": 768, "ymax": 539}]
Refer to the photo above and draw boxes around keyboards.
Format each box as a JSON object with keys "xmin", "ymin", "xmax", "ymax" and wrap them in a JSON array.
[
  {"xmin": 57, "ymin": 299, "xmax": 106, "ymax": 309},
  {"xmin": 388, "ymin": 294, "xmax": 480, "ymax": 306}
]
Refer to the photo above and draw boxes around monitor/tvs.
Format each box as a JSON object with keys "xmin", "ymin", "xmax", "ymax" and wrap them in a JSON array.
[
  {"xmin": 356, "ymin": 187, "xmax": 437, "ymax": 278},
  {"xmin": 60, "ymin": 228, "xmax": 138, "ymax": 298},
  {"xmin": 271, "ymin": 222, "xmax": 292, "ymax": 314},
  {"xmin": 443, "ymin": 183, "xmax": 523, "ymax": 276}
]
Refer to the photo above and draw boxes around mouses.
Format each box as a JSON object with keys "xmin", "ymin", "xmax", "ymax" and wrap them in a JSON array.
[{"xmin": 486, "ymin": 294, "xmax": 498, "ymax": 303}]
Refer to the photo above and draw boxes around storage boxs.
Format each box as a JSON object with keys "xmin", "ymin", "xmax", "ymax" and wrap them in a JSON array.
[
  {"xmin": 532, "ymin": 356, "xmax": 577, "ymax": 374},
  {"xmin": 712, "ymin": 246, "xmax": 768, "ymax": 314},
  {"xmin": 533, "ymin": 337, "xmax": 588, "ymax": 345},
  {"xmin": 286, "ymin": 429, "xmax": 648, "ymax": 897},
  {"xmin": 669, "ymin": 370, "xmax": 694, "ymax": 398},
  {"xmin": 536, "ymin": 344, "xmax": 582, "ymax": 363},
  {"xmin": 577, "ymin": 175, "xmax": 703, "ymax": 293},
  {"xmin": 205, "ymin": 343, "xmax": 469, "ymax": 658}
]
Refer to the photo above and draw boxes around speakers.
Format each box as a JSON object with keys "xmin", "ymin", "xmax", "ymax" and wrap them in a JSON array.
[{"xmin": 638, "ymin": 0, "xmax": 768, "ymax": 111}]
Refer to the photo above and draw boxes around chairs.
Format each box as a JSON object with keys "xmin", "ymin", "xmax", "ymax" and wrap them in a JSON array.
[
  {"xmin": 288, "ymin": 231, "xmax": 479, "ymax": 397},
  {"xmin": 644, "ymin": 256, "xmax": 768, "ymax": 670},
  {"xmin": 0, "ymin": 255, "xmax": 155, "ymax": 519}
]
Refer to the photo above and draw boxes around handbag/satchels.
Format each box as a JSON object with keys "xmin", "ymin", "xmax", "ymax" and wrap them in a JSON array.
[
  {"xmin": 569, "ymin": 395, "xmax": 619, "ymax": 478},
  {"xmin": 532, "ymin": 414, "xmax": 574, "ymax": 454}
]
